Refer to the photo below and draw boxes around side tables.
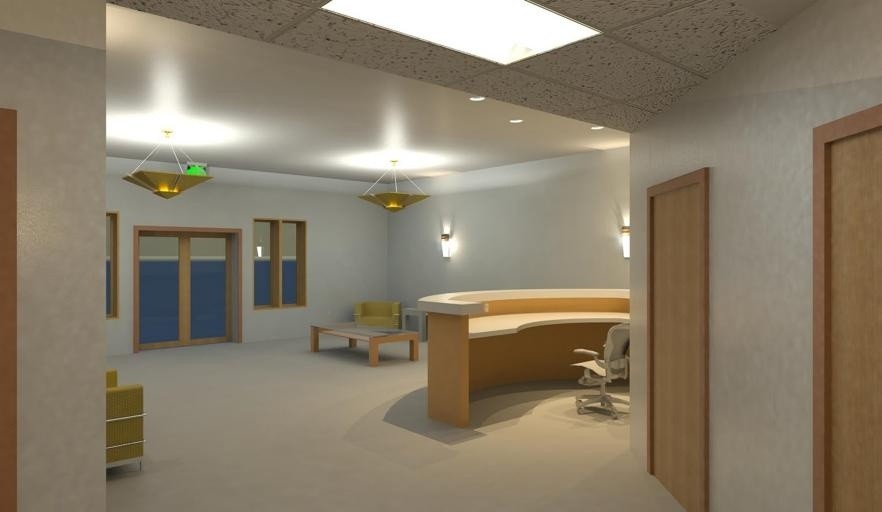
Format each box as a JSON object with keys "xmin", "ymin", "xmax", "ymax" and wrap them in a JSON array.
[{"xmin": 402, "ymin": 309, "xmax": 426, "ymax": 341}]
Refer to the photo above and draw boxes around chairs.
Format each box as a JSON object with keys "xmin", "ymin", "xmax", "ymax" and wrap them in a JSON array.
[
  {"xmin": 569, "ymin": 324, "xmax": 630, "ymax": 421},
  {"xmin": 354, "ymin": 301, "xmax": 401, "ymax": 332},
  {"xmin": 107, "ymin": 370, "xmax": 145, "ymax": 475}
]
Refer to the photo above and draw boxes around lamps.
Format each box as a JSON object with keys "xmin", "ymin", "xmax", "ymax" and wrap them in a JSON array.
[
  {"xmin": 620, "ymin": 226, "xmax": 631, "ymax": 258},
  {"xmin": 440, "ymin": 234, "xmax": 450, "ymax": 258},
  {"xmin": 122, "ymin": 132, "xmax": 214, "ymax": 200},
  {"xmin": 356, "ymin": 161, "xmax": 431, "ymax": 213}
]
